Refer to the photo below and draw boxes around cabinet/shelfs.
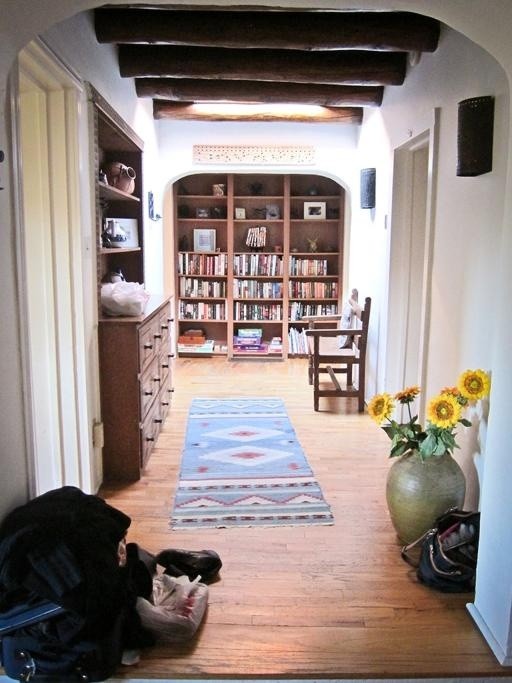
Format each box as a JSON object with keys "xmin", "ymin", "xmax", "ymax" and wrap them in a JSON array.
[
  {"xmin": 89, "ymin": 82, "xmax": 144, "ymax": 320},
  {"xmin": 174, "ymin": 173, "xmax": 345, "ymax": 360},
  {"xmin": 98, "ymin": 292, "xmax": 174, "ymax": 481}
]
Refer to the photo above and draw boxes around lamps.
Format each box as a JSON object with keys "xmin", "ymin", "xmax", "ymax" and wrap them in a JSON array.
[{"xmin": 192, "ymin": 103, "xmax": 320, "ymax": 116}]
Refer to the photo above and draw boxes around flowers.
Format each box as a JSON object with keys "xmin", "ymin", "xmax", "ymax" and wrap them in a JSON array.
[{"xmin": 367, "ymin": 369, "xmax": 490, "ymax": 457}]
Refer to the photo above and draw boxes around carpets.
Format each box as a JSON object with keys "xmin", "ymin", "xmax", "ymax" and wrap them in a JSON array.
[{"xmin": 171, "ymin": 397, "xmax": 335, "ymax": 530}]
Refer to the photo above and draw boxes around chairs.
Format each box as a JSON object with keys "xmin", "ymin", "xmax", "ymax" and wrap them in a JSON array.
[{"xmin": 302, "ymin": 288, "xmax": 371, "ymax": 412}]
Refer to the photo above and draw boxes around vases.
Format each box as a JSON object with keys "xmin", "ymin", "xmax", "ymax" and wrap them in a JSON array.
[{"xmin": 388, "ymin": 451, "xmax": 466, "ymax": 546}]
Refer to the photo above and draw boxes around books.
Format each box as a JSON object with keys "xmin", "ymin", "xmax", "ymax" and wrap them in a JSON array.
[
  {"xmin": 233, "ymin": 279, "xmax": 283, "ymax": 298},
  {"xmin": 177, "ymin": 329, "xmax": 227, "ymax": 353},
  {"xmin": 288, "ymin": 280, "xmax": 338, "ymax": 299},
  {"xmin": 288, "ymin": 327, "xmax": 309, "ymax": 354},
  {"xmin": 179, "ymin": 277, "xmax": 226, "ymax": 298},
  {"xmin": 233, "ymin": 254, "xmax": 284, "ymax": 276},
  {"xmin": 178, "ymin": 300, "xmax": 224, "ymax": 320},
  {"xmin": 288, "ymin": 256, "xmax": 327, "ymax": 276},
  {"xmin": 178, "ymin": 252, "xmax": 228, "ymax": 276},
  {"xmin": 234, "ymin": 302, "xmax": 283, "ymax": 320},
  {"xmin": 233, "ymin": 329, "xmax": 282, "ymax": 355},
  {"xmin": 288, "ymin": 302, "xmax": 337, "ymax": 321}
]
